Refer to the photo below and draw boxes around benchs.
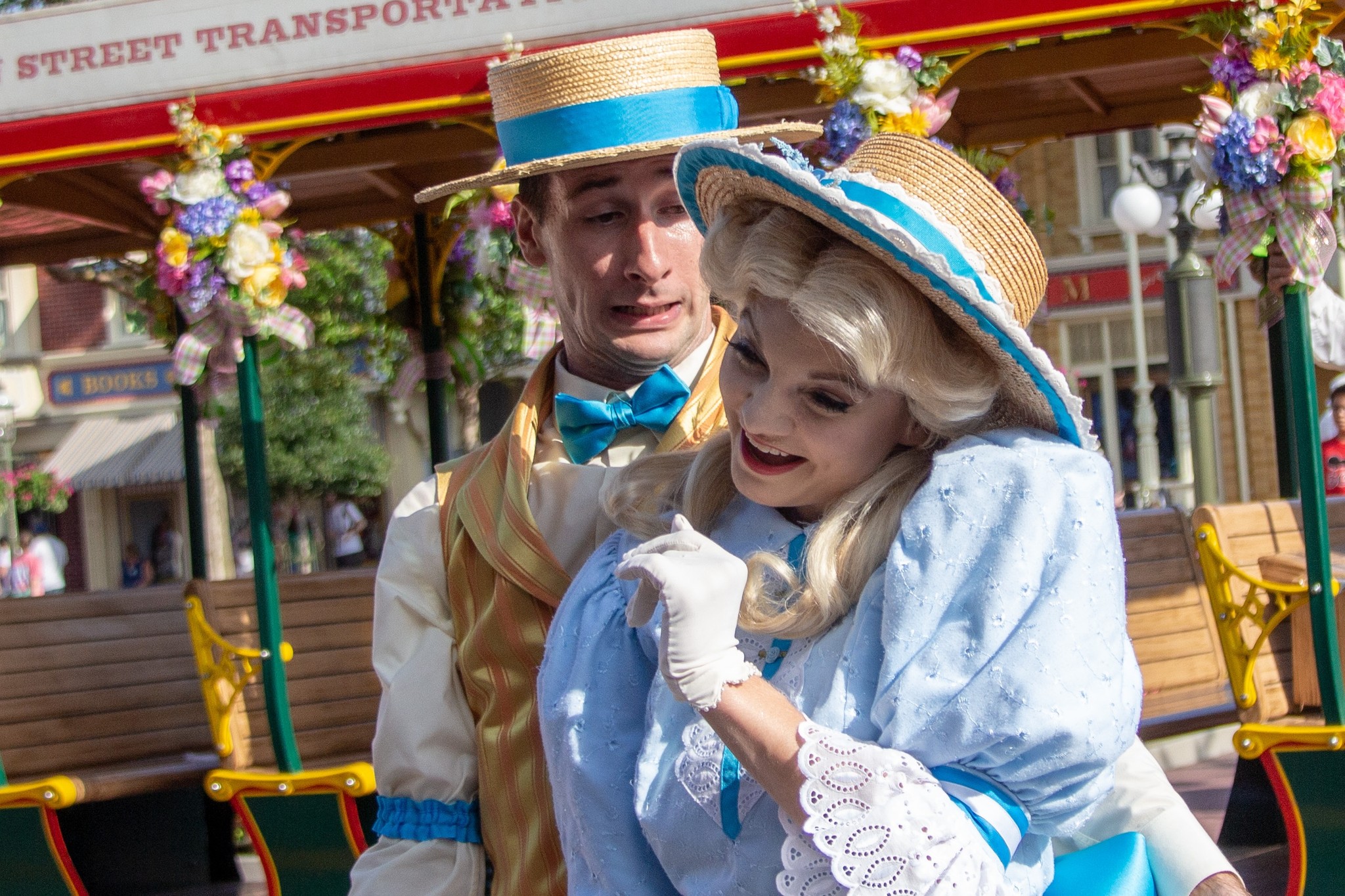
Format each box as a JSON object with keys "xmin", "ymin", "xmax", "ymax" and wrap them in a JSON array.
[
  {"xmin": 0, "ymin": 577, "xmax": 187, "ymax": 896},
  {"xmin": 1188, "ymin": 495, "xmax": 1345, "ymax": 896},
  {"xmin": 1115, "ymin": 502, "xmax": 1240, "ymax": 747},
  {"xmin": 189, "ymin": 563, "xmax": 379, "ymax": 896}
]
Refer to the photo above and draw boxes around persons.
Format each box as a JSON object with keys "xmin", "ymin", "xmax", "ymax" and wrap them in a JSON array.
[
  {"xmin": 536, "ymin": 132, "xmax": 1140, "ymax": 896},
  {"xmin": 230, "ymin": 476, "xmax": 387, "ymax": 577},
  {"xmin": 0, "ymin": 511, "xmax": 70, "ymax": 599},
  {"xmin": 1321, "ymin": 385, "xmax": 1345, "ymax": 496},
  {"xmin": 354, "ymin": 23, "xmax": 1250, "ymax": 896},
  {"xmin": 122, "ymin": 505, "xmax": 183, "ymax": 588}
]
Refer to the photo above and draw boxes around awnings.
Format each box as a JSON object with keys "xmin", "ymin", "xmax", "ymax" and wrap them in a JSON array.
[{"xmin": 43, "ymin": 414, "xmax": 186, "ymax": 489}]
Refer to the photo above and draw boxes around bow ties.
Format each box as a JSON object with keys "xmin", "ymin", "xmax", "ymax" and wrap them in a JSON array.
[{"xmin": 554, "ymin": 364, "xmax": 690, "ymax": 464}]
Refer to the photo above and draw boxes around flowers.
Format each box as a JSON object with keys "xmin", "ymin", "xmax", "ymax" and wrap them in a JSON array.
[
  {"xmin": 137, "ymin": 85, "xmax": 306, "ymax": 319},
  {"xmin": 1182, "ymin": 0, "xmax": 1345, "ymax": 298},
  {"xmin": 789, "ymin": 0, "xmax": 1056, "ymax": 230}
]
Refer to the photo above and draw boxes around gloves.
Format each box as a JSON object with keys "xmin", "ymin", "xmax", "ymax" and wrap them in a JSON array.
[{"xmin": 614, "ymin": 513, "xmax": 764, "ymax": 714}]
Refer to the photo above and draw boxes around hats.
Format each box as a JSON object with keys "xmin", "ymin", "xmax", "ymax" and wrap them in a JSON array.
[
  {"xmin": 672, "ymin": 133, "xmax": 1104, "ymax": 452},
  {"xmin": 414, "ymin": 29, "xmax": 823, "ymax": 206}
]
